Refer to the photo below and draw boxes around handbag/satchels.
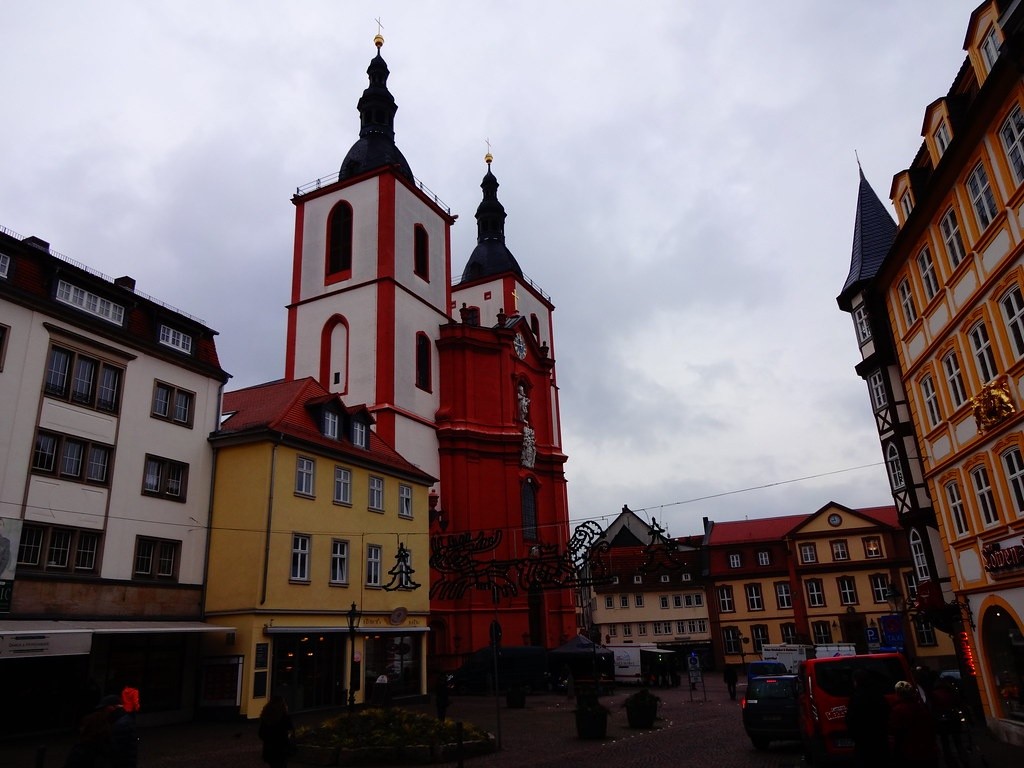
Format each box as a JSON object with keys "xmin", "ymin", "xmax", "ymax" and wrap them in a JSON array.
[{"xmin": 289, "ymin": 730, "xmax": 298, "ymax": 758}]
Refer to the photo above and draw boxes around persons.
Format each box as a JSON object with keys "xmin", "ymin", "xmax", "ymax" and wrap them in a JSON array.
[
  {"xmin": 64, "ymin": 692, "xmax": 137, "ymax": 768},
  {"xmin": 723, "ymin": 664, "xmax": 738, "ymax": 699},
  {"xmin": 844, "ymin": 667, "xmax": 973, "ymax": 768},
  {"xmin": 258, "ymin": 696, "xmax": 294, "ymax": 768}
]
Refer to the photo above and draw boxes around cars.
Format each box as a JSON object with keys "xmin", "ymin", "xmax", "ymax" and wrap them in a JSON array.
[{"xmin": 743, "ymin": 676, "xmax": 801, "ymax": 749}]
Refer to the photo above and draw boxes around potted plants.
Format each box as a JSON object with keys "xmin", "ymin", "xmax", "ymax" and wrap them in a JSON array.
[
  {"xmin": 571, "ymin": 681, "xmax": 613, "ymax": 741},
  {"xmin": 620, "ymin": 688, "xmax": 662, "ymax": 728}
]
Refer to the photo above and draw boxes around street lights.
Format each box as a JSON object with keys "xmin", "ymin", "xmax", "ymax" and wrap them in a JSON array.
[{"xmin": 345, "ymin": 601, "xmax": 362, "ymax": 709}]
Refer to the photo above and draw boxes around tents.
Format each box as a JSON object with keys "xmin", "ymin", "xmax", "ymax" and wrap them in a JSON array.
[{"xmin": 547, "ymin": 633, "xmax": 616, "ymax": 695}]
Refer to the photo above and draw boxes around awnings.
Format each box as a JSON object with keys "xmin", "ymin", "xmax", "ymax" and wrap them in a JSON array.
[
  {"xmin": 0, "ymin": 620, "xmax": 239, "ymax": 659},
  {"xmin": 641, "ymin": 648, "xmax": 693, "ymax": 668}
]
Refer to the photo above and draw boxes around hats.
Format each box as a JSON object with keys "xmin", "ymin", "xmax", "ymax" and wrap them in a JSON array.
[
  {"xmin": 894, "ymin": 680, "xmax": 913, "ymax": 697},
  {"xmin": 96, "ymin": 695, "xmax": 120, "ymax": 709}
]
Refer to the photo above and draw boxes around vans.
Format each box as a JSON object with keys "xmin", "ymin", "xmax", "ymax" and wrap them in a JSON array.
[
  {"xmin": 446, "ymin": 646, "xmax": 547, "ymax": 695},
  {"xmin": 747, "ymin": 661, "xmax": 787, "ymax": 683}
]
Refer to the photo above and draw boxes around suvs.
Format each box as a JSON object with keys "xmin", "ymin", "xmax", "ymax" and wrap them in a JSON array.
[{"xmin": 796, "ymin": 652, "xmax": 927, "ymax": 762}]
